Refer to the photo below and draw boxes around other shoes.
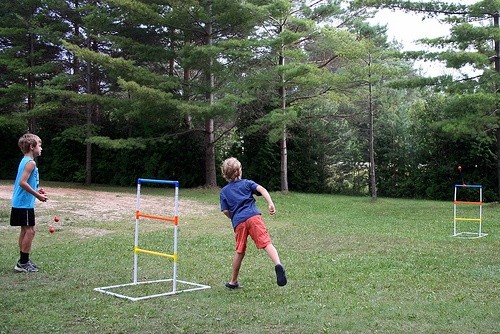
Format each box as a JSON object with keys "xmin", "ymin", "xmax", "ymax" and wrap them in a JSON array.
[
  {"xmin": 225, "ymin": 283, "xmax": 239, "ymax": 288},
  {"xmin": 275, "ymin": 264, "xmax": 287, "ymax": 286}
]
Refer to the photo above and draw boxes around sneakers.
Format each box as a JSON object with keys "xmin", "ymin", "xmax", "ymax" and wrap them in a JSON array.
[{"xmin": 15, "ymin": 261, "xmax": 38, "ymax": 272}]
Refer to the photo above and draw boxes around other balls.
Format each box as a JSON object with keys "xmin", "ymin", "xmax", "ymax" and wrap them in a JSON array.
[
  {"xmin": 53, "ymin": 216, "xmax": 59, "ymax": 222},
  {"xmin": 458, "ymin": 166, "xmax": 461, "ymax": 170},
  {"xmin": 49, "ymin": 227, "xmax": 54, "ymax": 233},
  {"xmin": 40, "ymin": 189, "xmax": 45, "ymax": 194}
]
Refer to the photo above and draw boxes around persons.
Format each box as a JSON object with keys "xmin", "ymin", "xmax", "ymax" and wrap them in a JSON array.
[
  {"xmin": 220, "ymin": 156, "xmax": 287, "ymax": 287},
  {"xmin": 10, "ymin": 134, "xmax": 48, "ymax": 271}
]
